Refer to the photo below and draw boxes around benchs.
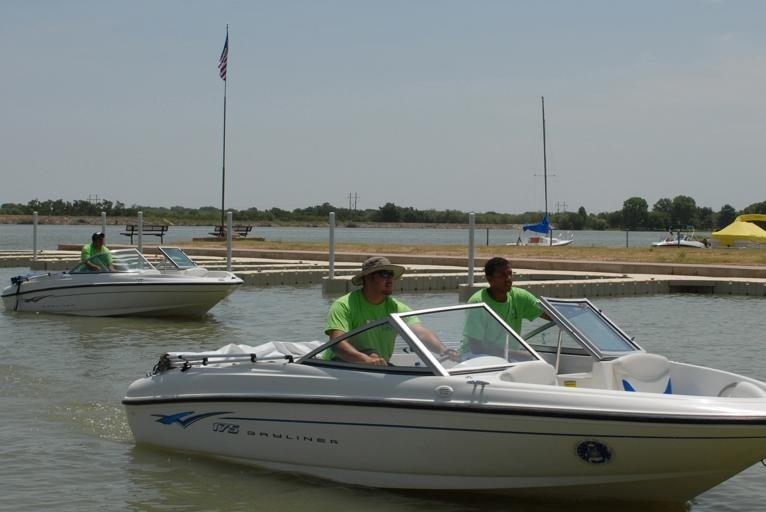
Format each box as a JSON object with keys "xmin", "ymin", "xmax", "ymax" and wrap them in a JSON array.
[
  {"xmin": 120, "ymin": 223, "xmax": 169, "ymax": 244},
  {"xmin": 208, "ymin": 224, "xmax": 254, "ymax": 239}
]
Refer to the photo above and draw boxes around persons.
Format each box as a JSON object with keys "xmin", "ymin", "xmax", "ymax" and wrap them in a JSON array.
[
  {"xmin": 321, "ymin": 255, "xmax": 464, "ymax": 371},
  {"xmin": 458, "ymin": 255, "xmax": 576, "ymax": 366},
  {"xmin": 78, "ymin": 231, "xmax": 117, "ymax": 273}
]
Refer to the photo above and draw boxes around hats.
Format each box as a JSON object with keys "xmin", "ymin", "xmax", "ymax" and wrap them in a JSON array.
[
  {"xmin": 350, "ymin": 256, "xmax": 407, "ymax": 287},
  {"xmin": 92, "ymin": 232, "xmax": 105, "ymax": 241}
]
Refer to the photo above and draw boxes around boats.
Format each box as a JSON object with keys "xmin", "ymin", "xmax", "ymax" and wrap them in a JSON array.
[
  {"xmin": 507, "ymin": 220, "xmax": 573, "ymax": 247},
  {"xmin": 122, "ymin": 296, "xmax": 766, "ymax": 504},
  {"xmin": 1, "ymin": 244, "xmax": 243, "ymax": 317},
  {"xmin": 653, "ymin": 224, "xmax": 704, "ymax": 247}
]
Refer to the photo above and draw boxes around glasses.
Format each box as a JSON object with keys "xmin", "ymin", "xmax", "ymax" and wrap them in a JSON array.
[{"xmin": 371, "ymin": 270, "xmax": 395, "ymax": 280}]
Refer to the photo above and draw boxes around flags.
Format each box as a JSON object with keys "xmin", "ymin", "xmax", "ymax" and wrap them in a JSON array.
[{"xmin": 216, "ymin": 23, "xmax": 228, "ymax": 83}]
